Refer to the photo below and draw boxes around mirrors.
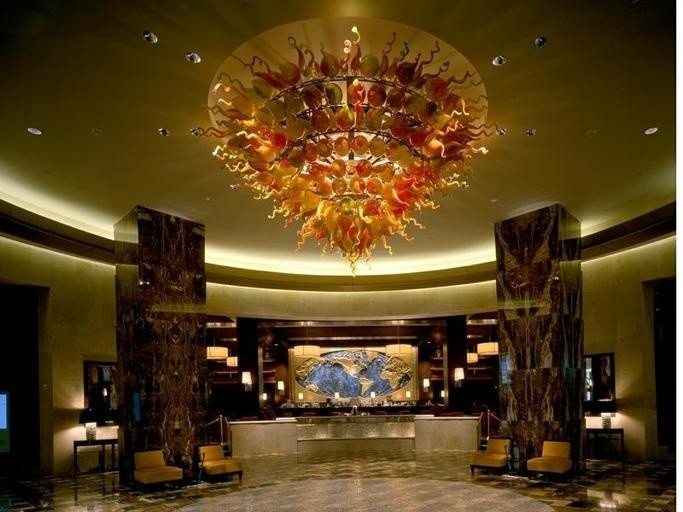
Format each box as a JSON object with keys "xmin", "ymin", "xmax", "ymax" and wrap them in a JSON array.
[
  {"xmin": 584, "ymin": 353, "xmax": 617, "ymax": 413},
  {"xmin": 84, "ymin": 360, "xmax": 120, "ymax": 421}
]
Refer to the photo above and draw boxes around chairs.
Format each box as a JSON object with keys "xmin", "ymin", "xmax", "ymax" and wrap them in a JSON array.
[
  {"xmin": 469, "ymin": 439, "xmax": 513, "ymax": 475},
  {"xmin": 131, "ymin": 450, "xmax": 183, "ymax": 491},
  {"xmin": 527, "ymin": 441, "xmax": 574, "ymax": 484},
  {"xmin": 194, "ymin": 445, "xmax": 243, "ymax": 483}
]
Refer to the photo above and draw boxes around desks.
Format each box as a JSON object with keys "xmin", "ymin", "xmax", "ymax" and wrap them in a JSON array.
[
  {"xmin": 73, "ymin": 439, "xmax": 119, "ymax": 474},
  {"xmin": 586, "ymin": 428, "xmax": 624, "ymax": 458}
]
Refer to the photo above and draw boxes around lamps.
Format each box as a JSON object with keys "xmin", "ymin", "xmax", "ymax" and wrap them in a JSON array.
[
  {"xmin": 191, "ymin": 26, "xmax": 504, "ymax": 275},
  {"xmin": 202, "ymin": 335, "xmax": 498, "ymax": 401}
]
